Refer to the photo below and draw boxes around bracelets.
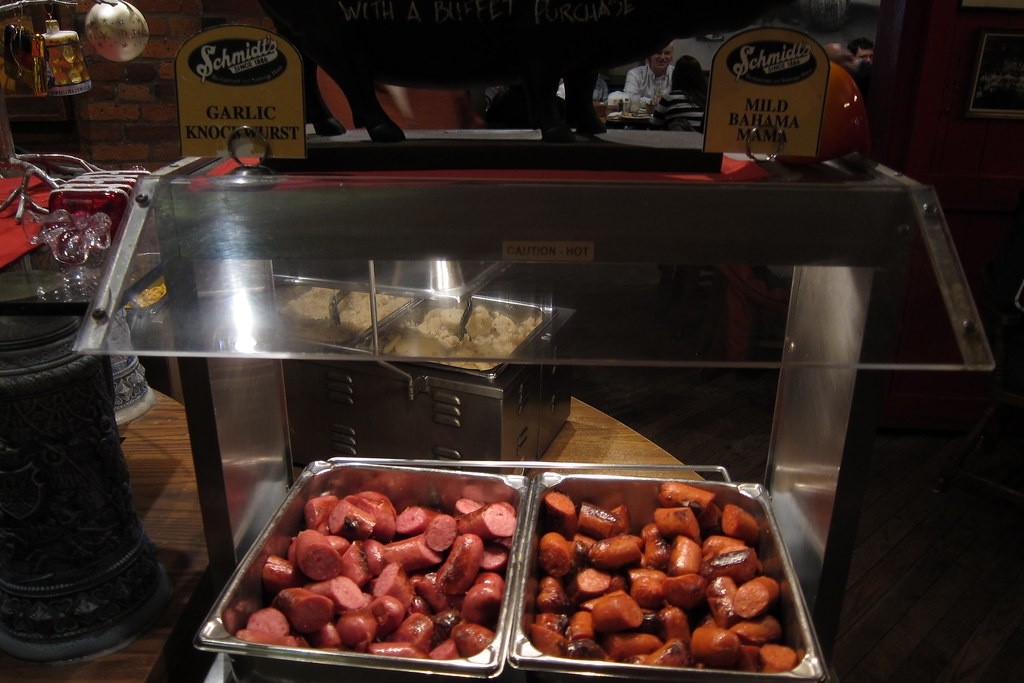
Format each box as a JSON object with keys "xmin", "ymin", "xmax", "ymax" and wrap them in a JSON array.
[{"xmin": 599, "ymin": 102, "xmax": 606, "ymax": 113}]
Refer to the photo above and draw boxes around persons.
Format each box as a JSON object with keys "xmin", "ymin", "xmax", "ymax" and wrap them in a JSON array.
[
  {"xmin": 558, "ymin": 44, "xmax": 703, "ymax": 133},
  {"xmin": 824, "ymin": 37, "xmax": 874, "ymax": 106}
]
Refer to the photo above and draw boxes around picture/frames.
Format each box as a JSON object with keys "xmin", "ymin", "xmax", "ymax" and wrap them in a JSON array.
[
  {"xmin": 959, "ymin": 23, "xmax": 1024, "ymax": 124},
  {"xmin": 956, "ymin": 0, "xmax": 1024, "ymax": 18}
]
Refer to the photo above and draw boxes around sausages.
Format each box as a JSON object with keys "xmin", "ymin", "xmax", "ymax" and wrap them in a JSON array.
[
  {"xmin": 233, "ymin": 491, "xmax": 515, "ymax": 660},
  {"xmin": 527, "ymin": 481, "xmax": 797, "ymax": 673}
]
